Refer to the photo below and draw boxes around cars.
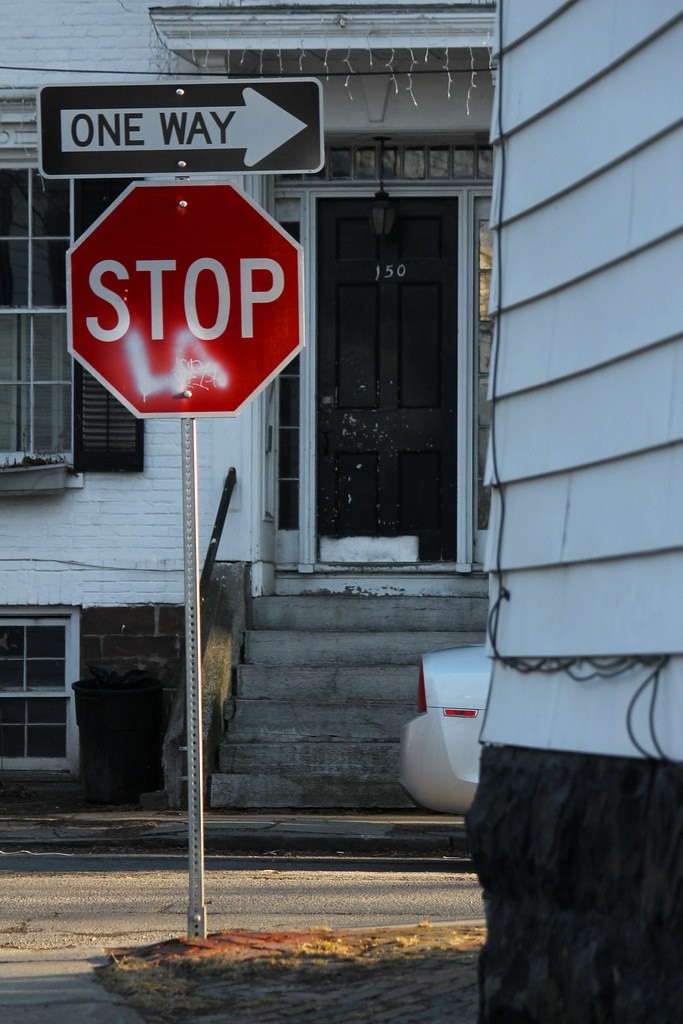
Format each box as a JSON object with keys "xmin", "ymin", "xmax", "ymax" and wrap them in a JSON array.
[{"xmin": 393, "ymin": 638, "xmax": 491, "ymax": 814}]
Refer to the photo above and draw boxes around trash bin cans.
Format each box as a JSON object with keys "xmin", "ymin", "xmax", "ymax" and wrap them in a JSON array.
[{"xmin": 71, "ymin": 678, "xmax": 164, "ymax": 806}]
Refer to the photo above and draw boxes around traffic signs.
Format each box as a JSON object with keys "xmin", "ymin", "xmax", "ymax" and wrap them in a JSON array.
[{"xmin": 35, "ymin": 77, "xmax": 326, "ymax": 181}]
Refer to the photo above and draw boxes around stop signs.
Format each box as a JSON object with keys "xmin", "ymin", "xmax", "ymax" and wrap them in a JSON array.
[{"xmin": 62, "ymin": 178, "xmax": 308, "ymax": 420}]
